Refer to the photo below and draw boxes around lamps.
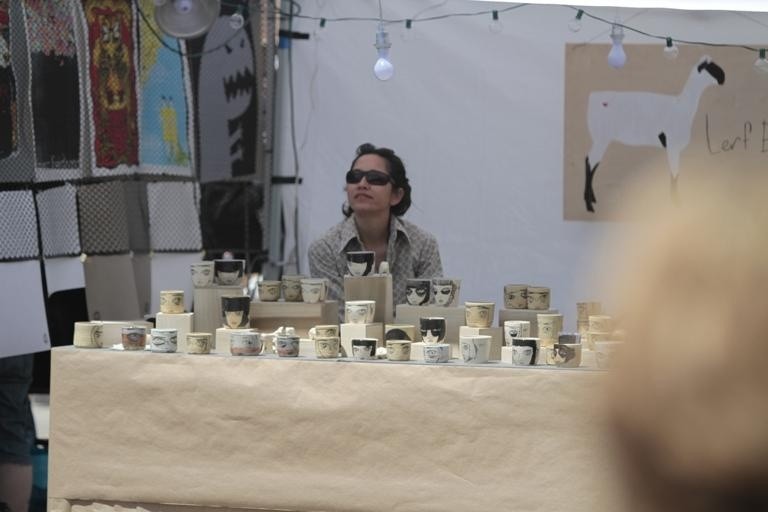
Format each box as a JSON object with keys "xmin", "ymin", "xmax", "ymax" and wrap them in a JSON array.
[{"xmin": 153, "ymin": 0, "xmax": 222, "ymax": 39}]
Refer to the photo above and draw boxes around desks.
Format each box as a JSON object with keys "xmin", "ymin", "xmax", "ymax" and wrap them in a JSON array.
[{"xmin": 47, "ymin": 336, "xmax": 603, "ymax": 511}]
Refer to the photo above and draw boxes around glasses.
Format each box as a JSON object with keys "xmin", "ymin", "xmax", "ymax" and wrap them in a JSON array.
[{"xmin": 346, "ymin": 169, "xmax": 395, "ymax": 185}]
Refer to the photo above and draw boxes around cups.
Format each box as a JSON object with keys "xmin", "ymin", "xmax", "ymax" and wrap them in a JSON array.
[
  {"xmin": 221, "ymin": 294, "xmax": 252, "ymax": 328},
  {"xmin": 190, "ymin": 258, "xmax": 247, "ymax": 287},
  {"xmin": 158, "ymin": 290, "xmax": 185, "ymax": 314},
  {"xmin": 344, "ymin": 299, "xmax": 376, "ymax": 324},
  {"xmin": 345, "ymin": 251, "xmax": 376, "ymax": 278},
  {"xmin": 73, "ymin": 317, "xmax": 629, "ymax": 370},
  {"xmin": 256, "ymin": 274, "xmax": 330, "ymax": 303},
  {"xmin": 503, "ymin": 283, "xmax": 553, "ymax": 310},
  {"xmin": 404, "ymin": 277, "xmax": 462, "ymax": 308},
  {"xmin": 575, "ymin": 300, "xmax": 602, "ymax": 332},
  {"xmin": 463, "ymin": 300, "xmax": 497, "ymax": 326}
]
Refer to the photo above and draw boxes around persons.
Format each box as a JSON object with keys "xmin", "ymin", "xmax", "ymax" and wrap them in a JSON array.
[
  {"xmin": 0, "ymin": 354, "xmax": 37, "ymax": 512},
  {"xmin": 308, "ymin": 144, "xmax": 444, "ymax": 325}
]
